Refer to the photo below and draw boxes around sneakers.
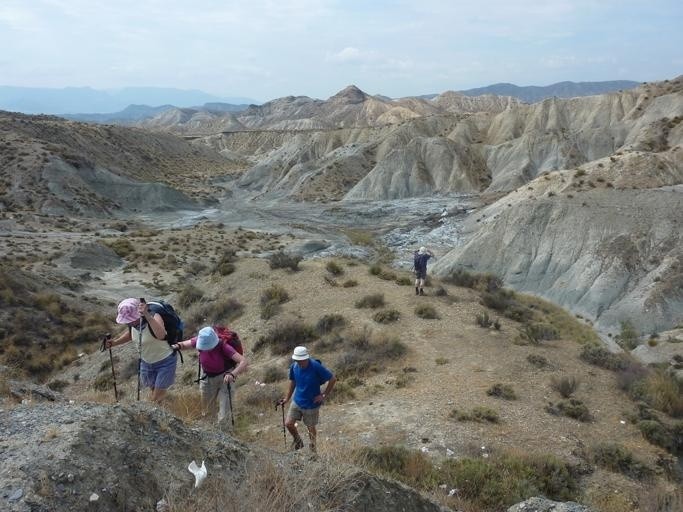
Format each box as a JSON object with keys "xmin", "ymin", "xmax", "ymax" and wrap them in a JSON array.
[
  {"xmin": 308, "ymin": 455, "xmax": 317, "ymax": 462},
  {"xmin": 290, "ymin": 439, "xmax": 303, "ymax": 451}
]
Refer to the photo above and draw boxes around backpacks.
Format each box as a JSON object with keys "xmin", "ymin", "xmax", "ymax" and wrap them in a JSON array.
[
  {"xmin": 129, "ymin": 300, "xmax": 183, "ymax": 344},
  {"xmin": 213, "ymin": 326, "xmax": 243, "ymax": 364}
]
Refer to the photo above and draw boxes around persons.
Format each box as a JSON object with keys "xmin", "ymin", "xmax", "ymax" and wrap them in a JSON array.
[
  {"xmin": 413, "ymin": 247, "xmax": 435, "ymax": 295},
  {"xmin": 279, "ymin": 346, "xmax": 336, "ymax": 462},
  {"xmin": 105, "ymin": 298, "xmax": 177, "ymax": 405},
  {"xmin": 170, "ymin": 326, "xmax": 247, "ymax": 434}
]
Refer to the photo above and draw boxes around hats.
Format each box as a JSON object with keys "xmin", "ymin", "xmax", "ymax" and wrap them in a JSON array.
[
  {"xmin": 418, "ymin": 247, "xmax": 426, "ymax": 255},
  {"xmin": 116, "ymin": 298, "xmax": 141, "ymax": 324},
  {"xmin": 292, "ymin": 346, "xmax": 309, "ymax": 361},
  {"xmin": 196, "ymin": 326, "xmax": 219, "ymax": 351}
]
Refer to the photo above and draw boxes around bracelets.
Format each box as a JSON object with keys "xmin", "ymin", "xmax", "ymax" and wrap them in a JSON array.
[
  {"xmin": 177, "ymin": 344, "xmax": 181, "ymax": 349},
  {"xmin": 322, "ymin": 393, "xmax": 327, "ymax": 399},
  {"xmin": 229, "ymin": 372, "xmax": 235, "ymax": 379}
]
